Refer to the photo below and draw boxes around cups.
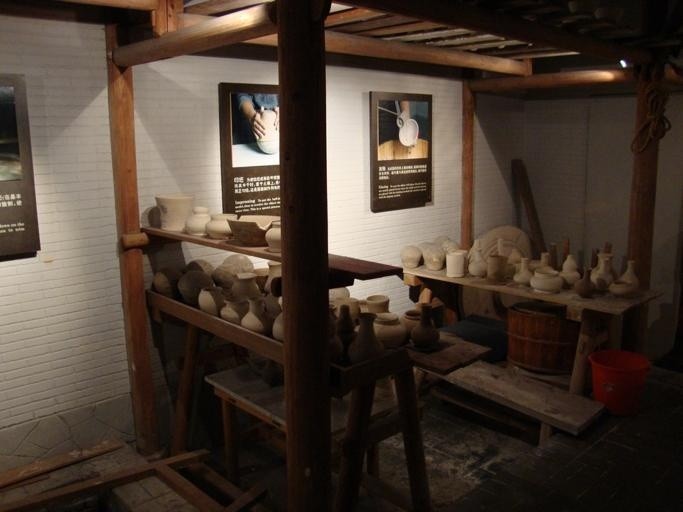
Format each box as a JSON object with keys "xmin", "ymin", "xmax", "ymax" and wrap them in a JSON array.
[{"xmin": 446, "ymin": 249, "xmax": 467, "ymax": 278}]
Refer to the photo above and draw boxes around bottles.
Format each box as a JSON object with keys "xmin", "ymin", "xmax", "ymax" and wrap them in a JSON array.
[
  {"xmin": 329, "ymin": 287, "xmax": 440, "ymax": 367},
  {"xmin": 468, "ymin": 238, "xmax": 533, "ymax": 288},
  {"xmin": 264, "ymin": 220, "xmax": 281, "ymax": 253},
  {"xmin": 184, "ymin": 206, "xmax": 239, "ymax": 241},
  {"xmin": 198, "ymin": 260, "xmax": 283, "ymax": 344},
  {"xmin": 530, "ymin": 231, "xmax": 640, "ymax": 301}
]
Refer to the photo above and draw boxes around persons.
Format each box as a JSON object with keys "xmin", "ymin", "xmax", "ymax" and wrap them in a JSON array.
[{"xmin": 236, "ymin": 92, "xmax": 281, "ymax": 141}]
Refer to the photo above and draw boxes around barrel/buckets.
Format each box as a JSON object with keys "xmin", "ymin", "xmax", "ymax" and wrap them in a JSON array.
[
  {"xmin": 587, "ymin": 346, "xmax": 650, "ymax": 417},
  {"xmin": 507, "ymin": 306, "xmax": 566, "ymax": 374}
]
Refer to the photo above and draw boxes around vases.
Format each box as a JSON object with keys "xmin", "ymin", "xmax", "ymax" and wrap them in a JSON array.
[
  {"xmin": 153, "ymin": 252, "xmax": 287, "ymax": 345},
  {"xmin": 153, "ymin": 190, "xmax": 283, "ymax": 253},
  {"xmin": 326, "ymin": 283, "xmax": 442, "ymax": 365},
  {"xmin": 400, "ymin": 232, "xmax": 642, "ymax": 298}
]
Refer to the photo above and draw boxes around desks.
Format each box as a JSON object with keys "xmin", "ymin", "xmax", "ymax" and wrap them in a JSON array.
[{"xmin": 204, "ymin": 364, "xmax": 399, "ymax": 512}]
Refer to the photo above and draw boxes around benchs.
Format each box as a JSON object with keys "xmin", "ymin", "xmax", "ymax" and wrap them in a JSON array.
[{"xmin": 413, "ymin": 360, "xmax": 606, "ymax": 444}]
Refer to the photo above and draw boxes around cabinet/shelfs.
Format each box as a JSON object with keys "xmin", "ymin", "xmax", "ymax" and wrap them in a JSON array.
[
  {"xmin": 399, "ymin": 264, "xmax": 664, "ymax": 396},
  {"xmin": 141, "ymin": 222, "xmax": 432, "ymax": 512}
]
